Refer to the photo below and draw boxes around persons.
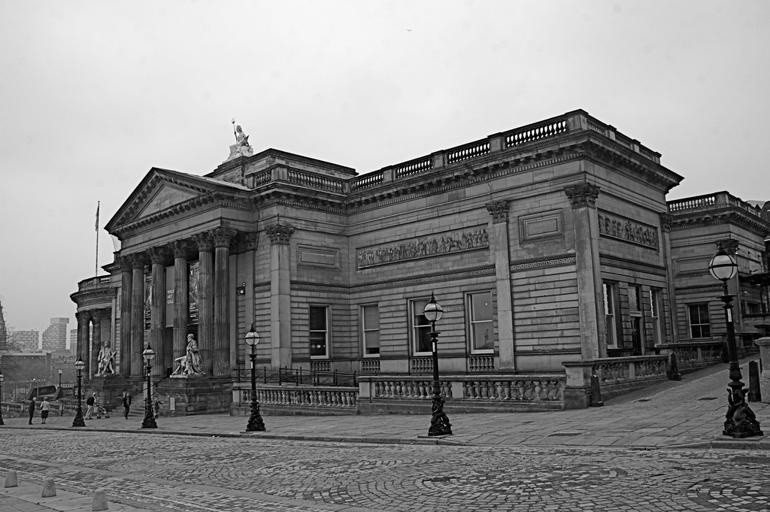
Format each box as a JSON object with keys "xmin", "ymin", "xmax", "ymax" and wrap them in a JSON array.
[
  {"xmin": 153, "ymin": 397, "xmax": 164, "ymax": 418},
  {"xmin": 27, "ymin": 396, "xmax": 37, "ymax": 425},
  {"xmin": 174, "ymin": 333, "xmax": 201, "ymax": 376},
  {"xmin": 84, "ymin": 392, "xmax": 97, "ymax": 420},
  {"xmin": 120, "ymin": 391, "xmax": 133, "ymax": 420},
  {"xmin": 226, "ymin": 125, "xmax": 250, "ymax": 160},
  {"xmin": 96, "ymin": 338, "xmax": 116, "ymax": 375},
  {"xmin": 39, "ymin": 396, "xmax": 51, "ymax": 424}
]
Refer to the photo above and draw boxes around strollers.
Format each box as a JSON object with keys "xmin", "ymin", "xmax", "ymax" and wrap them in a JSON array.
[{"xmin": 96, "ymin": 404, "xmax": 110, "ymax": 419}]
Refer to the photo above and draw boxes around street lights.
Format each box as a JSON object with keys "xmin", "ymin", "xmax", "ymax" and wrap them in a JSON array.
[
  {"xmin": 74, "ymin": 358, "xmax": 85, "ymax": 428},
  {"xmin": 141, "ymin": 342, "xmax": 158, "ymax": 429},
  {"xmin": 423, "ymin": 291, "xmax": 453, "ymax": 437},
  {"xmin": 0, "ymin": 373, "xmax": 5, "ymax": 425},
  {"xmin": 57, "ymin": 366, "xmax": 63, "ymax": 387},
  {"xmin": 243, "ymin": 323, "xmax": 267, "ymax": 432},
  {"xmin": 707, "ymin": 240, "xmax": 760, "ymax": 438}
]
